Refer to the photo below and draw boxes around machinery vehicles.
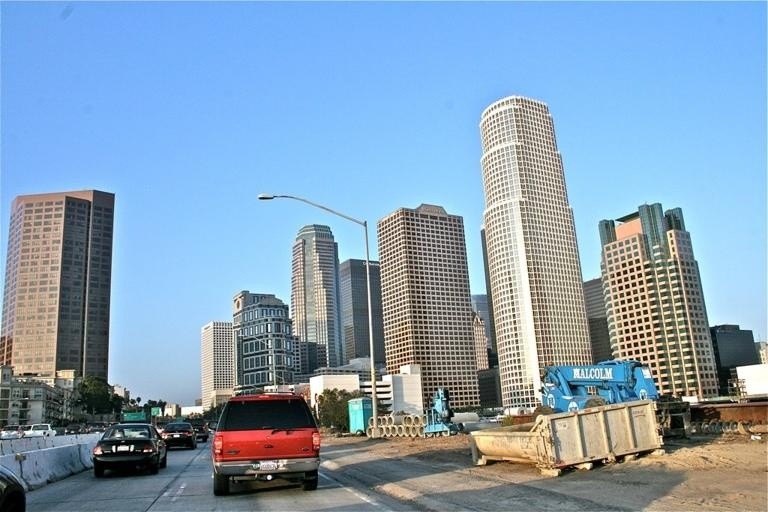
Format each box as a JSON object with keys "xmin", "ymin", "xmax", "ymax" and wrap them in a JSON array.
[
  {"xmin": 539, "ymin": 359, "xmax": 692, "ymax": 438},
  {"xmin": 422, "ymin": 386, "xmax": 457, "ymax": 436}
]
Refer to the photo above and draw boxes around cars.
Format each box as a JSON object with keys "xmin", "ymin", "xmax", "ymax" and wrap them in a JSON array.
[
  {"xmin": 208, "ymin": 391, "xmax": 321, "ymax": 496},
  {"xmin": 0, "ymin": 464, "xmax": 26, "ymax": 512},
  {"xmin": 0, "ymin": 421, "xmax": 57, "ymax": 440},
  {"xmin": 64, "ymin": 418, "xmax": 209, "ymax": 479}
]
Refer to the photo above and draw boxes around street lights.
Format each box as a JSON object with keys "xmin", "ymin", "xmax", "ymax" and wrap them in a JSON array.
[{"xmin": 257, "ymin": 192, "xmax": 379, "ymax": 439}]
